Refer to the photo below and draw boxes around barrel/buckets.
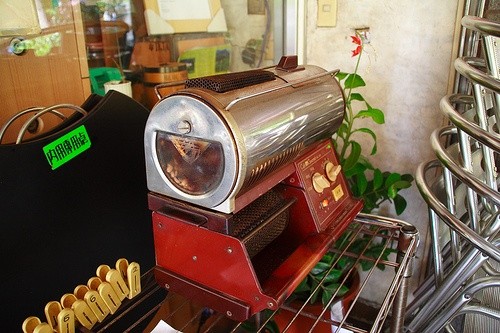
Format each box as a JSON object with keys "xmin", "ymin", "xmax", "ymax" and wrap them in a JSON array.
[{"xmin": 144, "ymin": 61, "xmax": 187, "ymax": 111}]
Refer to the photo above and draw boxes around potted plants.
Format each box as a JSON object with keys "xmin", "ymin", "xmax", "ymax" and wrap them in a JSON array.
[{"xmin": 266, "ymin": 27, "xmax": 417, "ymax": 333}]
